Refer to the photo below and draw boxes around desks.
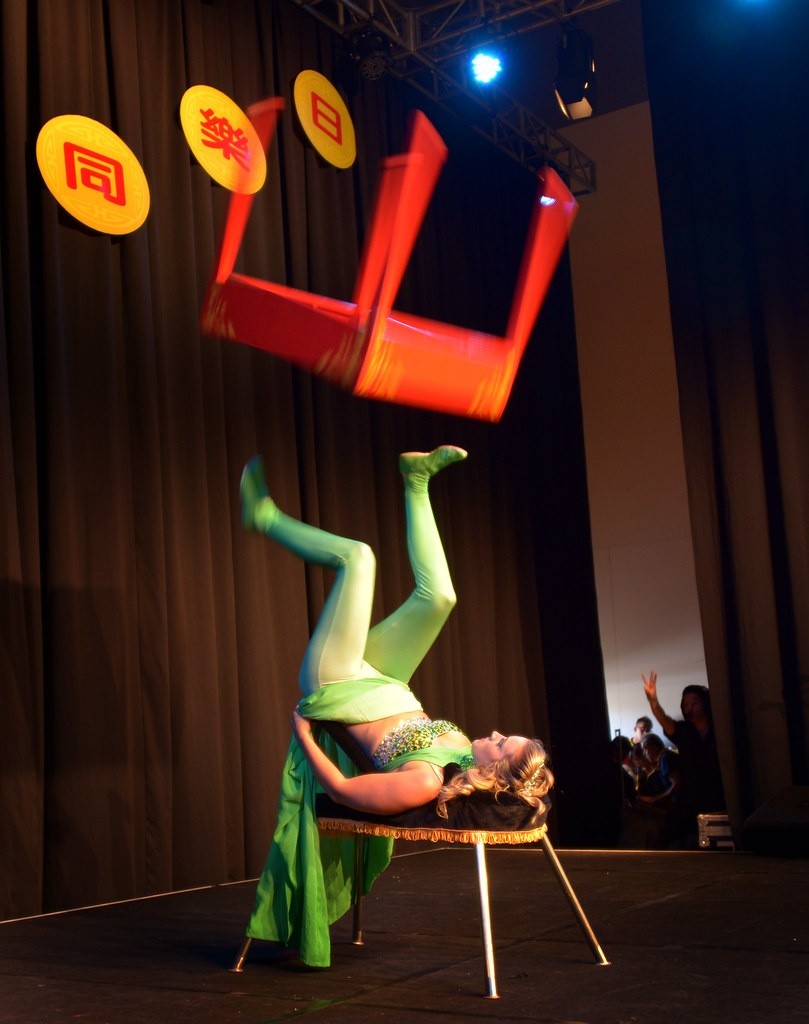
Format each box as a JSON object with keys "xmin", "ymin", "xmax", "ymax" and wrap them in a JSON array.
[
  {"xmin": 227, "ymin": 782, "xmax": 614, "ymax": 1002},
  {"xmin": 194, "ymin": 90, "xmax": 581, "ymax": 426}
]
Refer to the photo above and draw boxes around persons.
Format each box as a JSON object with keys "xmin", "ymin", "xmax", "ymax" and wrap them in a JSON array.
[
  {"xmin": 615, "ymin": 672, "xmax": 725, "ymax": 839},
  {"xmin": 227, "ymin": 444, "xmax": 554, "ymax": 834}
]
